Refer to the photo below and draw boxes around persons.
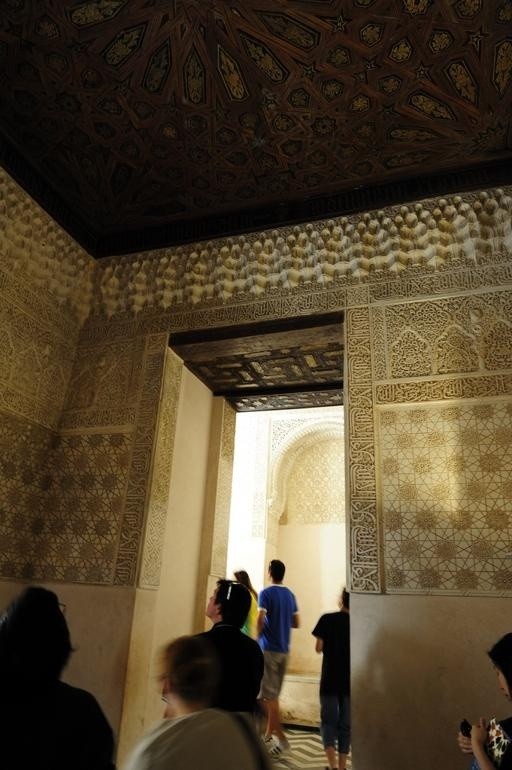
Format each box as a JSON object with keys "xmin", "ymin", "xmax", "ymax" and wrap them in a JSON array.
[
  {"xmin": 1, "ymin": 586, "xmax": 116, "ymax": 770},
  {"xmin": 160, "ymin": 578, "xmax": 265, "ymax": 721},
  {"xmin": 310, "ymin": 587, "xmax": 351, "ymax": 770},
  {"xmin": 457, "ymin": 631, "xmax": 511, "ymax": 770},
  {"xmin": 256, "ymin": 558, "xmax": 300, "ymax": 759},
  {"xmin": 124, "ymin": 634, "xmax": 274, "ymax": 770},
  {"xmin": 233, "ymin": 570, "xmax": 258, "ymax": 642}
]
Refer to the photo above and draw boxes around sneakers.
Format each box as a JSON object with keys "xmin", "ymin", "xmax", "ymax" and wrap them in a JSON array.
[{"xmin": 262, "ymin": 736, "xmax": 291, "ymax": 759}]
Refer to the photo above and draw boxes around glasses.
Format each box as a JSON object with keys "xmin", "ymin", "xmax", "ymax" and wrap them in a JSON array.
[
  {"xmin": 226, "ymin": 581, "xmax": 242, "ymax": 600},
  {"xmin": 58, "ymin": 601, "xmax": 67, "ymax": 615}
]
